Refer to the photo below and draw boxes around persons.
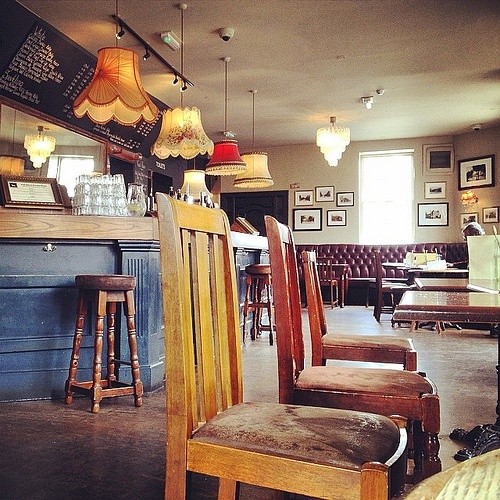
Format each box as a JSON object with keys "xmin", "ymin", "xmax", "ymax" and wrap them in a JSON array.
[{"xmin": 447, "ymin": 222, "xmax": 485, "ymax": 268}]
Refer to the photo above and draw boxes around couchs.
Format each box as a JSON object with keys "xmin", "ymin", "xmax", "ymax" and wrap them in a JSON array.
[{"xmin": 293, "ymin": 242, "xmax": 467, "ymax": 305}]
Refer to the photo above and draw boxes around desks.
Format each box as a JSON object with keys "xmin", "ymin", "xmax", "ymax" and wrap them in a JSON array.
[
  {"xmin": 316, "ymin": 263, "xmax": 349, "ymax": 308},
  {"xmin": 382, "ymin": 262, "xmax": 500, "ymax": 461}
]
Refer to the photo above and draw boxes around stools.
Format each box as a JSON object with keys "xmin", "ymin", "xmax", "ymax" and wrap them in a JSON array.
[
  {"xmin": 65, "ymin": 275, "xmax": 144, "ymax": 413},
  {"xmin": 241, "ymin": 263, "xmax": 276, "ymax": 346}
]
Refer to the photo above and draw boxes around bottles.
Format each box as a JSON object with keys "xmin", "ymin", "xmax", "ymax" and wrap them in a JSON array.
[{"xmin": 167, "ymin": 186, "xmax": 182, "ymax": 200}]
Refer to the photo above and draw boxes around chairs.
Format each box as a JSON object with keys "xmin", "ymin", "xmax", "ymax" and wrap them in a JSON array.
[
  {"xmin": 301, "ymin": 251, "xmax": 417, "ymax": 459},
  {"xmin": 263, "ymin": 215, "xmax": 442, "ymax": 485},
  {"xmin": 373, "ymin": 251, "xmax": 410, "ymax": 320},
  {"xmin": 156, "ymin": 192, "xmax": 407, "ymax": 500}
]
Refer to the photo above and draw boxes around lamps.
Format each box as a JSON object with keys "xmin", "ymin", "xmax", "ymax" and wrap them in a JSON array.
[
  {"xmin": 25, "ymin": 126, "xmax": 56, "ymax": 168},
  {"xmin": 461, "ymin": 191, "xmax": 477, "ymax": 204},
  {"xmin": 72, "ymin": 1, "xmax": 275, "ymax": 199},
  {"xmin": 316, "ymin": 117, "xmax": 350, "ymax": 167}
]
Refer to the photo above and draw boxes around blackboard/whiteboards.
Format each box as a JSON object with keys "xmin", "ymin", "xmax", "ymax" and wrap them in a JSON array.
[{"xmin": 1, "ymin": 0, "xmax": 174, "ymax": 160}]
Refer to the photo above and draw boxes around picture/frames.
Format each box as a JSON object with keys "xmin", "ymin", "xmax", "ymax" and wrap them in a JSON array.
[
  {"xmin": 292, "ymin": 185, "xmax": 354, "ymax": 231},
  {"xmin": 418, "ymin": 144, "xmax": 500, "ymax": 231}
]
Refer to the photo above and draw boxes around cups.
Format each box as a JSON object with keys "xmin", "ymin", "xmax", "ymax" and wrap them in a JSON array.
[
  {"xmin": 126, "ymin": 183, "xmax": 147, "ymax": 217},
  {"xmin": 72, "ymin": 173, "xmax": 128, "ymax": 217}
]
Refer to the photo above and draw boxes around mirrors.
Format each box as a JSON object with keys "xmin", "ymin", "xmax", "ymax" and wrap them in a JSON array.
[{"xmin": 0, "ymin": 95, "xmax": 108, "ymax": 198}]
